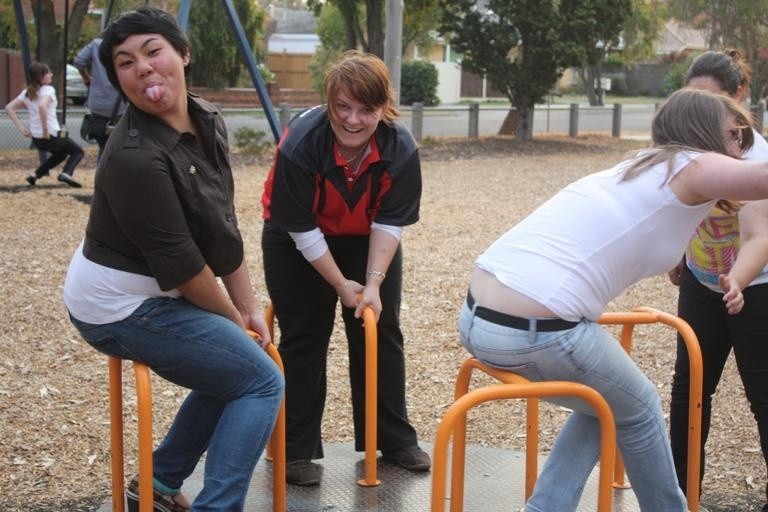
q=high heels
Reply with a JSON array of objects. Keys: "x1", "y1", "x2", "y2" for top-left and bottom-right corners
[{"x1": 127, "y1": 473, "x2": 190, "y2": 512}]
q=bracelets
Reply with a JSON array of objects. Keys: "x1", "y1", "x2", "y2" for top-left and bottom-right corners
[{"x1": 365, "y1": 269, "x2": 386, "y2": 282}]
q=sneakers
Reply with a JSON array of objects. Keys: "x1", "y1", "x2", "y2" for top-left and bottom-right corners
[
  {"x1": 286, "y1": 459, "x2": 320, "y2": 486},
  {"x1": 380, "y1": 446, "x2": 431, "y2": 469}
]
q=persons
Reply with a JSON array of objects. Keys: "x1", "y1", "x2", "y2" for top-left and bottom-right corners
[
  {"x1": 667, "y1": 47, "x2": 768, "y2": 512},
  {"x1": 456, "y1": 87, "x2": 768, "y2": 512},
  {"x1": 5, "y1": 61, "x2": 85, "y2": 189},
  {"x1": 61, "y1": 7, "x2": 287, "y2": 512},
  {"x1": 259, "y1": 48, "x2": 432, "y2": 484},
  {"x1": 73, "y1": 37, "x2": 129, "y2": 161}
]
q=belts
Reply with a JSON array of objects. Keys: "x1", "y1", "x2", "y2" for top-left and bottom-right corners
[{"x1": 466, "y1": 289, "x2": 581, "y2": 332}]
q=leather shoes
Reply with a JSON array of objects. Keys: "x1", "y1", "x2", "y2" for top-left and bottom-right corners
[
  {"x1": 26, "y1": 175, "x2": 36, "y2": 185},
  {"x1": 58, "y1": 174, "x2": 82, "y2": 187}
]
